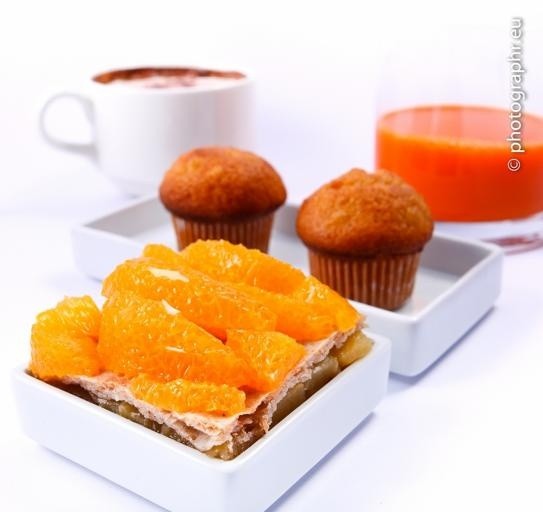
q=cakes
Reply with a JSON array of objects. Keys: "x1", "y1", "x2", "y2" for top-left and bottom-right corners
[
  {"x1": 158, "y1": 146, "x2": 287, "y2": 255},
  {"x1": 30, "y1": 239, "x2": 372, "y2": 460},
  {"x1": 295, "y1": 168, "x2": 434, "y2": 311}
]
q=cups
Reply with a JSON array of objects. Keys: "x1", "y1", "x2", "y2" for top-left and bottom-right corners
[{"x1": 38, "y1": 63, "x2": 256, "y2": 197}]
